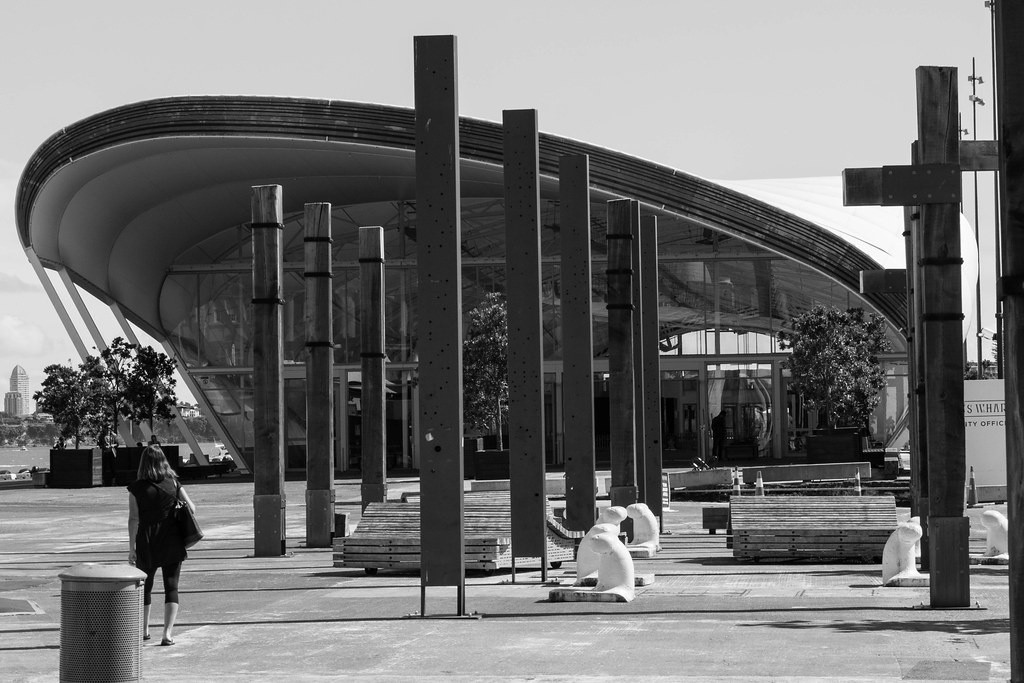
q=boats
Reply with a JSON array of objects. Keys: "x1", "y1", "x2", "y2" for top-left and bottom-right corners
[
  {"x1": 215, "y1": 443, "x2": 230, "y2": 455},
  {"x1": 20, "y1": 446, "x2": 27, "y2": 451}
]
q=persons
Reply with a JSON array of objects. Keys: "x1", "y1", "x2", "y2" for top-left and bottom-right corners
[
  {"x1": 64, "y1": 439, "x2": 67, "y2": 448},
  {"x1": 886, "y1": 415, "x2": 895, "y2": 433},
  {"x1": 53, "y1": 437, "x2": 64, "y2": 450},
  {"x1": 136, "y1": 442, "x2": 142, "y2": 447},
  {"x1": 148, "y1": 435, "x2": 160, "y2": 446},
  {"x1": 712, "y1": 410, "x2": 727, "y2": 460},
  {"x1": 104, "y1": 436, "x2": 118, "y2": 448},
  {"x1": 127, "y1": 444, "x2": 195, "y2": 646}
]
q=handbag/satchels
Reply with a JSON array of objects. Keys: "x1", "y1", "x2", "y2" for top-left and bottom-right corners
[{"x1": 175, "y1": 499, "x2": 204, "y2": 549}]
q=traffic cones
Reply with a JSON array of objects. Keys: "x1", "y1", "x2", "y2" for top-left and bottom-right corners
[
  {"x1": 731, "y1": 466, "x2": 742, "y2": 497},
  {"x1": 967, "y1": 465, "x2": 983, "y2": 509},
  {"x1": 852, "y1": 466, "x2": 863, "y2": 497},
  {"x1": 755, "y1": 470, "x2": 764, "y2": 496}
]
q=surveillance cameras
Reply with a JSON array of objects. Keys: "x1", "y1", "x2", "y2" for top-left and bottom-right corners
[{"x1": 980, "y1": 328, "x2": 996, "y2": 340}]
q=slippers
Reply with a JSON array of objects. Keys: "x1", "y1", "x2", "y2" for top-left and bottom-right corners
[
  {"x1": 161, "y1": 640, "x2": 175, "y2": 645},
  {"x1": 143, "y1": 634, "x2": 151, "y2": 639}
]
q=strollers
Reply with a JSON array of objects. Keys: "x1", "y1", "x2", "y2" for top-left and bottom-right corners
[{"x1": 690, "y1": 456, "x2": 717, "y2": 471}]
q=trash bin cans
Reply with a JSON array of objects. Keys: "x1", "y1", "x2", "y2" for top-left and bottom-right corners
[{"x1": 57, "y1": 565, "x2": 147, "y2": 683}]
